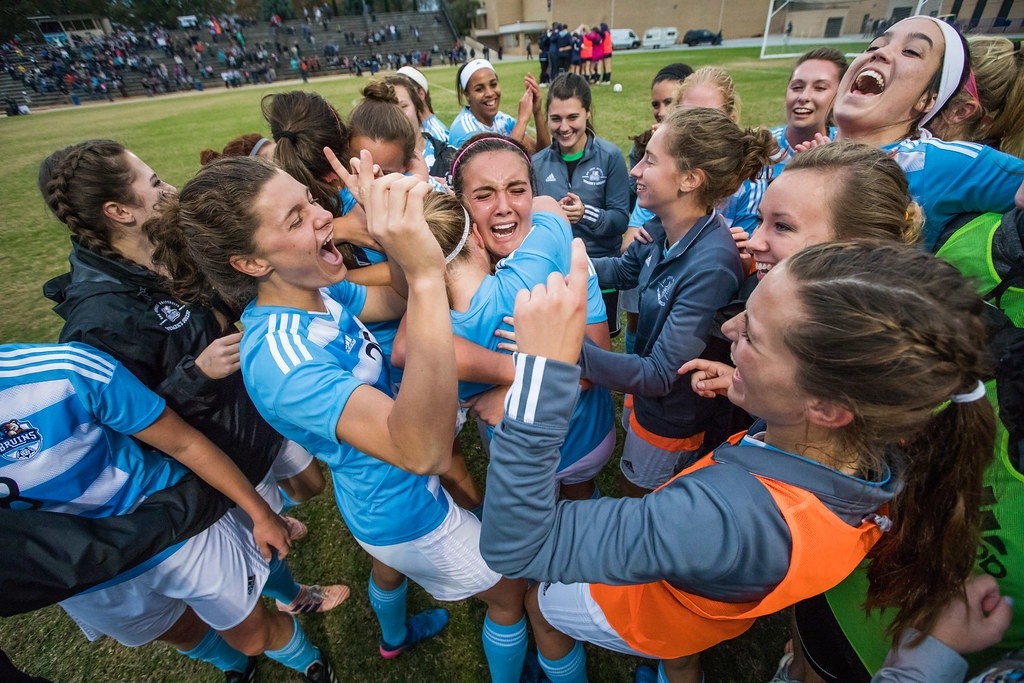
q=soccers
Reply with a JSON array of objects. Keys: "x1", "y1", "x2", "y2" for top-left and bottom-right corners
[{"x1": 613, "y1": 84, "x2": 622, "y2": 92}]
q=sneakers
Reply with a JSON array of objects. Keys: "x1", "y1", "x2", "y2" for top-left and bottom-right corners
[
  {"x1": 275, "y1": 584, "x2": 350, "y2": 615},
  {"x1": 224, "y1": 655, "x2": 257, "y2": 683},
  {"x1": 302, "y1": 647, "x2": 339, "y2": 683},
  {"x1": 279, "y1": 515, "x2": 307, "y2": 539},
  {"x1": 379, "y1": 608, "x2": 448, "y2": 660}
]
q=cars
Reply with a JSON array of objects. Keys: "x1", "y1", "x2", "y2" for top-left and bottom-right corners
[{"x1": 682, "y1": 28, "x2": 718, "y2": 47}]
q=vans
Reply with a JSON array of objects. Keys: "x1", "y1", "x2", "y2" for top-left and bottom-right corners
[
  {"x1": 609, "y1": 28, "x2": 641, "y2": 50},
  {"x1": 643, "y1": 26, "x2": 677, "y2": 48}
]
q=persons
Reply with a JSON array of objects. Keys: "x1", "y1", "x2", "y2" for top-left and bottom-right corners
[
  {"x1": 0, "y1": 0, "x2": 475, "y2": 117},
  {"x1": 478, "y1": 14, "x2": 1024, "y2": 683},
  {"x1": 0, "y1": 140, "x2": 350, "y2": 682},
  {"x1": 141, "y1": 59, "x2": 629, "y2": 683}
]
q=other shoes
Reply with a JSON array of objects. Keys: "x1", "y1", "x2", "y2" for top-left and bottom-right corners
[
  {"x1": 635, "y1": 665, "x2": 658, "y2": 683},
  {"x1": 519, "y1": 652, "x2": 540, "y2": 683}
]
